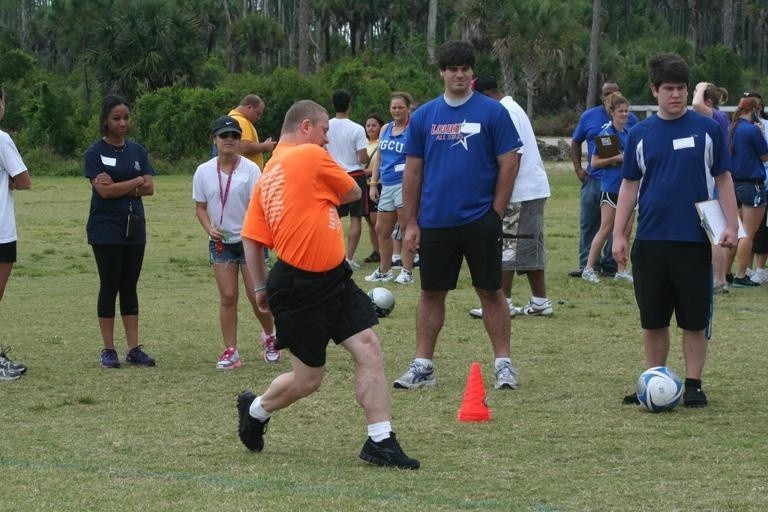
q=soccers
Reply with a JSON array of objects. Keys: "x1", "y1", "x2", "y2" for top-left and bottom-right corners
[
  {"x1": 367, "y1": 287, "x2": 395, "y2": 318},
  {"x1": 637, "y1": 365, "x2": 683, "y2": 413}
]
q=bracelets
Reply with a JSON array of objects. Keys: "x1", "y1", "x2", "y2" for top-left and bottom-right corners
[{"x1": 135, "y1": 187, "x2": 139, "y2": 197}]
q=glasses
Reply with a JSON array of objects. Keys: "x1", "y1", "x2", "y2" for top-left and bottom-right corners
[{"x1": 215, "y1": 133, "x2": 240, "y2": 140}]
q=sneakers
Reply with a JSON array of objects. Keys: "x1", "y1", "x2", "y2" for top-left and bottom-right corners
[
  {"x1": 393, "y1": 360, "x2": 436, "y2": 389},
  {"x1": 0, "y1": 345, "x2": 27, "y2": 374},
  {"x1": 215, "y1": 347, "x2": 241, "y2": 369},
  {"x1": 358, "y1": 431, "x2": 420, "y2": 471},
  {"x1": 99, "y1": 348, "x2": 120, "y2": 368},
  {"x1": 623, "y1": 392, "x2": 640, "y2": 406},
  {"x1": 712, "y1": 267, "x2": 768, "y2": 294},
  {"x1": 515, "y1": 299, "x2": 554, "y2": 317},
  {"x1": 236, "y1": 390, "x2": 271, "y2": 453},
  {"x1": 262, "y1": 330, "x2": 281, "y2": 364},
  {"x1": 349, "y1": 250, "x2": 420, "y2": 286},
  {"x1": 568, "y1": 269, "x2": 633, "y2": 286},
  {"x1": 470, "y1": 298, "x2": 516, "y2": 319},
  {"x1": 124, "y1": 344, "x2": 156, "y2": 367},
  {"x1": 682, "y1": 383, "x2": 708, "y2": 408},
  {"x1": 0, "y1": 364, "x2": 20, "y2": 380}
]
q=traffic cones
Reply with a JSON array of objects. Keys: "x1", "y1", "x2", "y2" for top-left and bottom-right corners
[{"x1": 457, "y1": 361, "x2": 490, "y2": 422}]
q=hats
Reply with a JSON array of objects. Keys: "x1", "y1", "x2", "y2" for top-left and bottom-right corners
[
  {"x1": 470, "y1": 75, "x2": 497, "y2": 91},
  {"x1": 210, "y1": 115, "x2": 243, "y2": 137}
]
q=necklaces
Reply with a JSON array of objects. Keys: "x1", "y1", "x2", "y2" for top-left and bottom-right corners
[{"x1": 112, "y1": 144, "x2": 127, "y2": 153}]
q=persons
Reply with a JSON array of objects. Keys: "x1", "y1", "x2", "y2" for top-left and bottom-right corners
[
  {"x1": 612, "y1": 56, "x2": 738, "y2": 407},
  {"x1": 0, "y1": 88, "x2": 32, "y2": 379},
  {"x1": 325, "y1": 40, "x2": 554, "y2": 390},
  {"x1": 85, "y1": 96, "x2": 156, "y2": 369},
  {"x1": 691, "y1": 81, "x2": 767, "y2": 293},
  {"x1": 194, "y1": 96, "x2": 280, "y2": 370},
  {"x1": 237, "y1": 101, "x2": 420, "y2": 469},
  {"x1": 568, "y1": 79, "x2": 641, "y2": 283}
]
